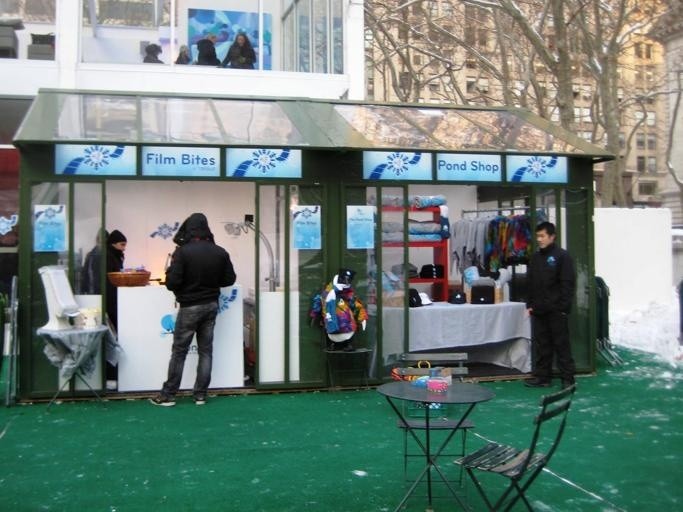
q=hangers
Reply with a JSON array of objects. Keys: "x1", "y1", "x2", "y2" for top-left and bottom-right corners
[{"x1": 460, "y1": 209, "x2": 545, "y2": 217}]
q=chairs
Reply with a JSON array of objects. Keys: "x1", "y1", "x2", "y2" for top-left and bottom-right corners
[
  {"x1": 453, "y1": 382, "x2": 577, "y2": 512},
  {"x1": 397, "y1": 352, "x2": 475, "y2": 511},
  {"x1": 322, "y1": 302, "x2": 372, "y2": 394}
]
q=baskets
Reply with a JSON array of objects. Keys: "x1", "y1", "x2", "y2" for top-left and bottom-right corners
[{"x1": 107, "y1": 270, "x2": 150, "y2": 286}]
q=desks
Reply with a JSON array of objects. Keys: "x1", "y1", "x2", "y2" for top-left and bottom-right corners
[
  {"x1": 36, "y1": 324, "x2": 111, "y2": 412},
  {"x1": 375, "y1": 378, "x2": 496, "y2": 512},
  {"x1": 365, "y1": 300, "x2": 533, "y2": 373}
]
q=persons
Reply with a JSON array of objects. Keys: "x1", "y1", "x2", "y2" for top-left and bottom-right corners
[
  {"x1": 523, "y1": 222, "x2": 575, "y2": 392},
  {"x1": 175, "y1": 45, "x2": 190, "y2": 64},
  {"x1": 82, "y1": 231, "x2": 127, "y2": 391},
  {"x1": 216, "y1": 34, "x2": 257, "y2": 68},
  {"x1": 143, "y1": 44, "x2": 165, "y2": 63},
  {"x1": 151, "y1": 212, "x2": 237, "y2": 406},
  {"x1": 197, "y1": 36, "x2": 221, "y2": 65}
]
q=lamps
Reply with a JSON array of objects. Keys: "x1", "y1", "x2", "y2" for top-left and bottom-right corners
[{"x1": 224, "y1": 221, "x2": 275, "y2": 291}]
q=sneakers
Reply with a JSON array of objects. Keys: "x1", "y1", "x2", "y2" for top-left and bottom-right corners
[
  {"x1": 192, "y1": 396, "x2": 205, "y2": 405},
  {"x1": 150, "y1": 396, "x2": 176, "y2": 406}
]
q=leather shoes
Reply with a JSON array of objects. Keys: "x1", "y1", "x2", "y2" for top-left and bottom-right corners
[
  {"x1": 561, "y1": 378, "x2": 575, "y2": 393},
  {"x1": 522, "y1": 377, "x2": 551, "y2": 387}
]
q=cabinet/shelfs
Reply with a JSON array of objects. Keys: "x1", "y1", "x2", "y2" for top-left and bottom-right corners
[
  {"x1": 376, "y1": 203, "x2": 449, "y2": 303},
  {"x1": 244, "y1": 288, "x2": 299, "y2": 383}
]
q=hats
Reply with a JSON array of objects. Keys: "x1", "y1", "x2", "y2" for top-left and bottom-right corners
[
  {"x1": 447, "y1": 292, "x2": 465, "y2": 304},
  {"x1": 339, "y1": 269, "x2": 355, "y2": 278},
  {"x1": 417, "y1": 292, "x2": 432, "y2": 305},
  {"x1": 108, "y1": 230, "x2": 125, "y2": 244}
]
